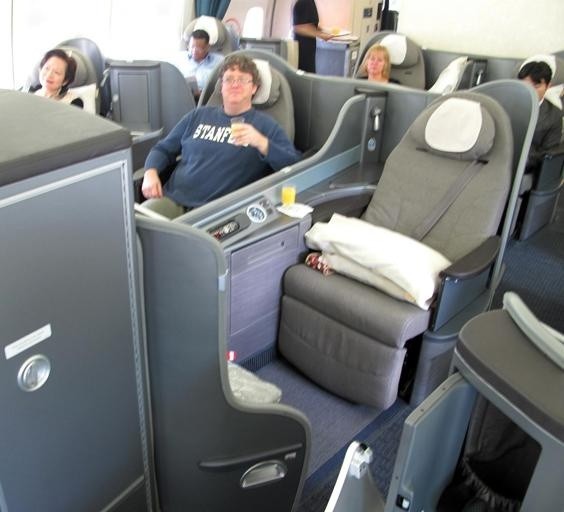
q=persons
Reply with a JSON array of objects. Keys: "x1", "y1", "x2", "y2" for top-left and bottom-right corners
[
  {"x1": 290, "y1": 0, "x2": 335, "y2": 74},
  {"x1": 356, "y1": 43, "x2": 404, "y2": 85},
  {"x1": 168, "y1": 28, "x2": 225, "y2": 106},
  {"x1": 518, "y1": 60, "x2": 564, "y2": 172},
  {"x1": 22, "y1": 47, "x2": 85, "y2": 108},
  {"x1": 141, "y1": 54, "x2": 304, "y2": 220}
]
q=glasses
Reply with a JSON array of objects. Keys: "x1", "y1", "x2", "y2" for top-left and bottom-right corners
[{"x1": 224, "y1": 77, "x2": 253, "y2": 85}]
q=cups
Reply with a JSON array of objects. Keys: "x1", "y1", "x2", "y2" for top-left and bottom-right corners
[
  {"x1": 281, "y1": 181, "x2": 296, "y2": 208},
  {"x1": 230, "y1": 117, "x2": 245, "y2": 142}
]
